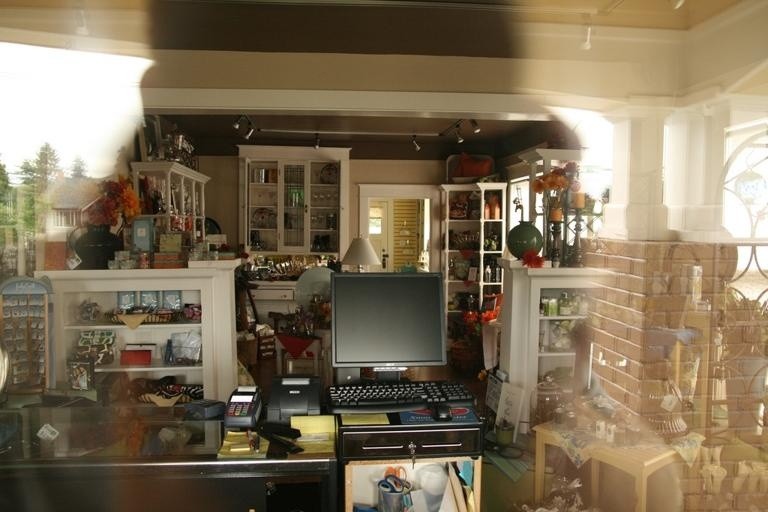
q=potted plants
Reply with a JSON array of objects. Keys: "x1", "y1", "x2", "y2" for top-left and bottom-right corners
[{"x1": 716, "y1": 287, "x2": 768, "y2": 432}]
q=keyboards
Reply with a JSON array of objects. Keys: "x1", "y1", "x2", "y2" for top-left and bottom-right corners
[{"x1": 325, "y1": 379, "x2": 477, "y2": 414}]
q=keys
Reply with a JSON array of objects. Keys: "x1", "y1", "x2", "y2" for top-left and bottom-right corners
[{"x1": 410, "y1": 441, "x2": 416, "y2": 467}]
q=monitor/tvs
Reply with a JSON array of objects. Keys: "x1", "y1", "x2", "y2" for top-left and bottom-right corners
[{"x1": 331, "y1": 272, "x2": 447, "y2": 384}]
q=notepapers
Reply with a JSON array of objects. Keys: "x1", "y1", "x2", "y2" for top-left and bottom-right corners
[{"x1": 230, "y1": 444, "x2": 251, "y2": 452}]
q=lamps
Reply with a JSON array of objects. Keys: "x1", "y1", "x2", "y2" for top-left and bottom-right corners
[
  {"x1": 441, "y1": 118, "x2": 481, "y2": 145},
  {"x1": 258, "y1": 129, "x2": 441, "y2": 152},
  {"x1": 233, "y1": 114, "x2": 256, "y2": 140},
  {"x1": 71, "y1": 8, "x2": 94, "y2": 44},
  {"x1": 579, "y1": 20, "x2": 593, "y2": 52}
]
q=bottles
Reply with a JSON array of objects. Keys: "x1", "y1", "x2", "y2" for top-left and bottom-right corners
[
  {"x1": 507, "y1": 221, "x2": 545, "y2": 259},
  {"x1": 310, "y1": 234, "x2": 337, "y2": 252},
  {"x1": 472, "y1": 256, "x2": 502, "y2": 283},
  {"x1": 484, "y1": 194, "x2": 500, "y2": 219},
  {"x1": 542, "y1": 289, "x2": 596, "y2": 316},
  {"x1": 484, "y1": 226, "x2": 502, "y2": 251},
  {"x1": 251, "y1": 231, "x2": 266, "y2": 252}
]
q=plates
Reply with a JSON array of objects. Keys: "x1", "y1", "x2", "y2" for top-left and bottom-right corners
[
  {"x1": 253, "y1": 208, "x2": 271, "y2": 228},
  {"x1": 321, "y1": 164, "x2": 336, "y2": 185}
]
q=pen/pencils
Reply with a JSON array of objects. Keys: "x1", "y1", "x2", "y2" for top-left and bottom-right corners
[
  {"x1": 379, "y1": 482, "x2": 414, "y2": 512},
  {"x1": 248, "y1": 429, "x2": 259, "y2": 453}
]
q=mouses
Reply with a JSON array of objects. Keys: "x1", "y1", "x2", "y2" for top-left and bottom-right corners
[{"x1": 433, "y1": 405, "x2": 453, "y2": 422}]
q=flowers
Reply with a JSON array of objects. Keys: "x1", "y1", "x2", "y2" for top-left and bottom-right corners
[{"x1": 514, "y1": 489, "x2": 590, "y2": 512}]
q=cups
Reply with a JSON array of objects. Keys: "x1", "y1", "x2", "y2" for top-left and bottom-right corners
[{"x1": 191, "y1": 238, "x2": 219, "y2": 260}]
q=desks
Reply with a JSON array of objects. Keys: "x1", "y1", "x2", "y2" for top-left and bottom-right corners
[
  {"x1": 0, "y1": 405, "x2": 339, "y2": 512},
  {"x1": 532, "y1": 421, "x2": 701, "y2": 512}
]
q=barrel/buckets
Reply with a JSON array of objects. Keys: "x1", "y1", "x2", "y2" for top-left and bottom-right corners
[{"x1": 378, "y1": 479, "x2": 412, "y2": 511}]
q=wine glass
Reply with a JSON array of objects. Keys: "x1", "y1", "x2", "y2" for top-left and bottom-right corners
[
  {"x1": 311, "y1": 189, "x2": 339, "y2": 206},
  {"x1": 311, "y1": 212, "x2": 326, "y2": 230}
]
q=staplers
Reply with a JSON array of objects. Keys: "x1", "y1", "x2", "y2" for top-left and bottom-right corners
[{"x1": 263, "y1": 424, "x2": 304, "y2": 455}]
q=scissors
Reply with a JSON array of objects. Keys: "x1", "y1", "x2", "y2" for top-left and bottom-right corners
[
  {"x1": 384, "y1": 467, "x2": 407, "y2": 483},
  {"x1": 378, "y1": 475, "x2": 403, "y2": 493}
]
